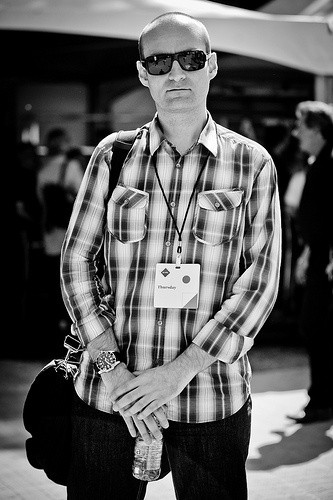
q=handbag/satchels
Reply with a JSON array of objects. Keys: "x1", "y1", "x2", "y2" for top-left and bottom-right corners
[
  {"x1": 21, "y1": 354, "x2": 84, "y2": 485},
  {"x1": 40, "y1": 180, "x2": 78, "y2": 231}
]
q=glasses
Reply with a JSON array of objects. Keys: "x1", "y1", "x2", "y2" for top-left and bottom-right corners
[{"x1": 141, "y1": 50, "x2": 212, "y2": 76}]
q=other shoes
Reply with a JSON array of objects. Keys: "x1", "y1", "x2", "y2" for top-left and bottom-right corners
[{"x1": 286, "y1": 406, "x2": 330, "y2": 425}]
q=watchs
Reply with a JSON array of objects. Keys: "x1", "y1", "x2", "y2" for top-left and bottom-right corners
[{"x1": 93, "y1": 351, "x2": 123, "y2": 374}]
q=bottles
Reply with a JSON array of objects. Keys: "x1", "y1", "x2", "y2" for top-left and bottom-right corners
[{"x1": 132, "y1": 404, "x2": 167, "y2": 481}]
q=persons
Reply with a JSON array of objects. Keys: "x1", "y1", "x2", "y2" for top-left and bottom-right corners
[
  {"x1": 280, "y1": 101, "x2": 333, "y2": 426},
  {"x1": 22, "y1": 11, "x2": 282, "y2": 500},
  {"x1": 0, "y1": 105, "x2": 307, "y2": 359}
]
